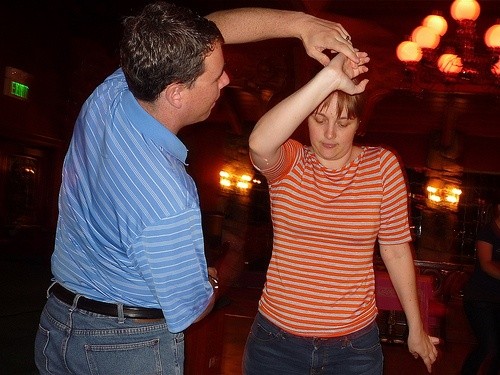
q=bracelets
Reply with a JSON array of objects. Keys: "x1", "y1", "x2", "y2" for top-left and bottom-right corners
[{"x1": 208, "y1": 274, "x2": 220, "y2": 290}]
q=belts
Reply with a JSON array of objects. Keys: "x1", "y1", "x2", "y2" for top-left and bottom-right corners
[{"x1": 50, "y1": 280, "x2": 165, "y2": 319}]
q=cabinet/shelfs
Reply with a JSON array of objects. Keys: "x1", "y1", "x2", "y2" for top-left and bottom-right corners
[{"x1": 371, "y1": 266, "x2": 443, "y2": 347}]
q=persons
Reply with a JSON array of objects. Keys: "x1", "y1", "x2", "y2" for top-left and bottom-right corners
[
  {"x1": 35, "y1": 0, "x2": 354, "y2": 375},
  {"x1": 243, "y1": 46, "x2": 440, "y2": 375},
  {"x1": 458, "y1": 202, "x2": 499, "y2": 375}
]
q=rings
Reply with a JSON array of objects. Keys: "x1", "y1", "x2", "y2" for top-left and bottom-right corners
[{"x1": 346, "y1": 36, "x2": 349, "y2": 41}]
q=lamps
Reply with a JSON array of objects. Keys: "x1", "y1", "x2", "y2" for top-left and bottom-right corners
[{"x1": 395, "y1": 0, "x2": 500, "y2": 80}]
